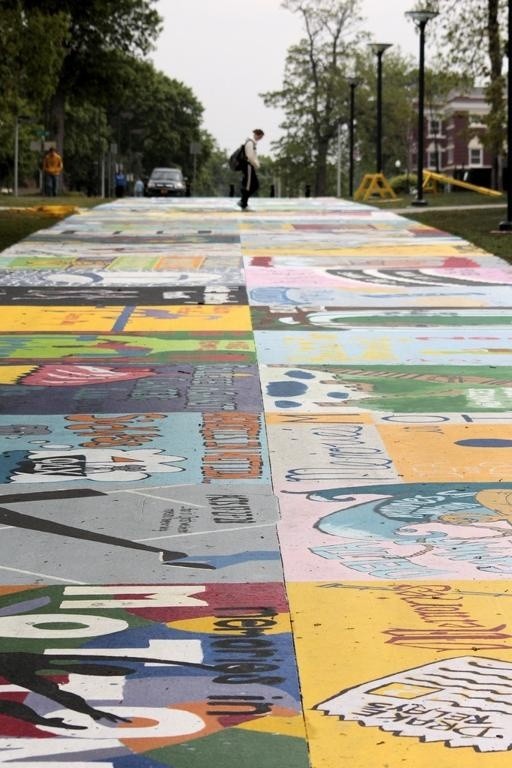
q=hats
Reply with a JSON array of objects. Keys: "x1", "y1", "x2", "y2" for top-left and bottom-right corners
[{"x1": 252, "y1": 127, "x2": 264, "y2": 135}]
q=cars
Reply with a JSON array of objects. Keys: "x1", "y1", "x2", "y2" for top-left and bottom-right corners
[{"x1": 145, "y1": 167, "x2": 189, "y2": 197}]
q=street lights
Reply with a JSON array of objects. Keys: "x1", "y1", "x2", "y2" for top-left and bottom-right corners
[
  {"x1": 368, "y1": 43, "x2": 392, "y2": 196},
  {"x1": 342, "y1": 74, "x2": 364, "y2": 196},
  {"x1": 405, "y1": 10, "x2": 437, "y2": 208}
]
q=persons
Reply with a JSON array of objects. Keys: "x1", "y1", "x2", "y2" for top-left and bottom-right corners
[
  {"x1": 114, "y1": 167, "x2": 128, "y2": 199},
  {"x1": 86, "y1": 154, "x2": 100, "y2": 198},
  {"x1": 133, "y1": 177, "x2": 145, "y2": 196},
  {"x1": 236, "y1": 129, "x2": 265, "y2": 212},
  {"x1": 42, "y1": 146, "x2": 64, "y2": 198}
]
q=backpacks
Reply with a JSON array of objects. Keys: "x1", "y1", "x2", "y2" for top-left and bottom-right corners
[{"x1": 228, "y1": 138, "x2": 255, "y2": 172}]
